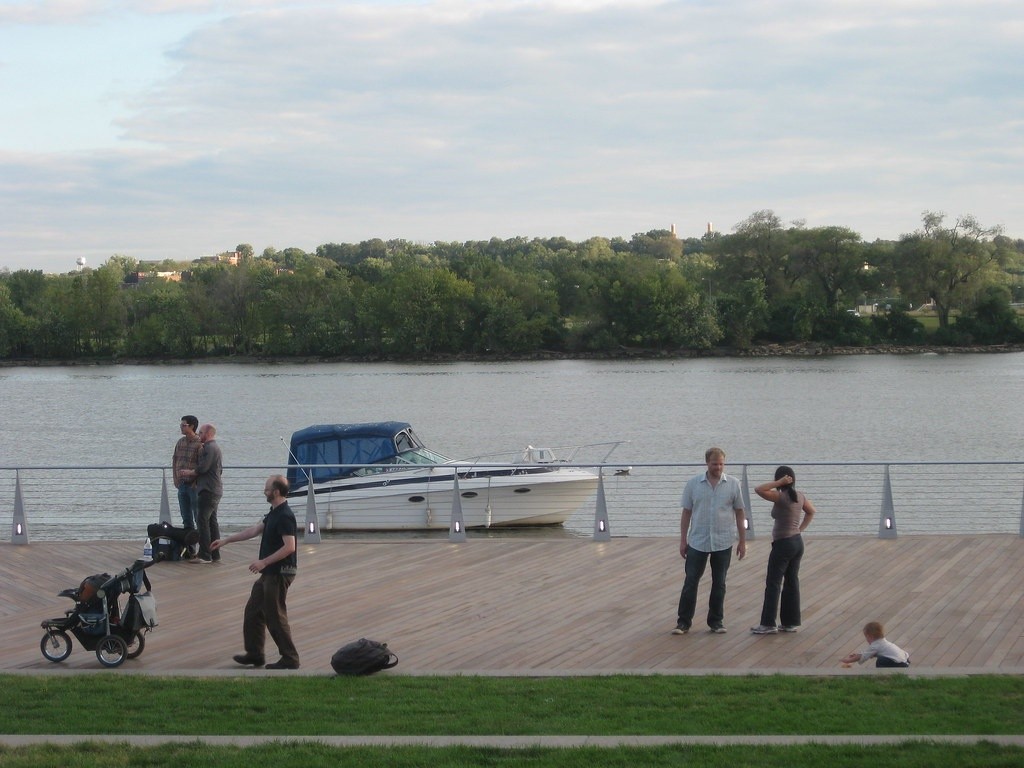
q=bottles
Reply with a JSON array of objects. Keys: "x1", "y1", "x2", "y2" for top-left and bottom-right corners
[{"x1": 144, "y1": 539, "x2": 152, "y2": 559}]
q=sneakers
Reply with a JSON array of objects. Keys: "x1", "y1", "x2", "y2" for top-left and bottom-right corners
[
  {"x1": 750, "y1": 625, "x2": 778, "y2": 633},
  {"x1": 778, "y1": 625, "x2": 797, "y2": 632},
  {"x1": 711, "y1": 626, "x2": 727, "y2": 633},
  {"x1": 671, "y1": 624, "x2": 689, "y2": 634}
]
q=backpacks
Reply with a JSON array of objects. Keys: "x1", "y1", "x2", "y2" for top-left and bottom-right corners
[{"x1": 330, "y1": 638, "x2": 399, "y2": 675}]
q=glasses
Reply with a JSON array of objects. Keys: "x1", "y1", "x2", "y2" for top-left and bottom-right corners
[{"x1": 180, "y1": 423, "x2": 191, "y2": 427}]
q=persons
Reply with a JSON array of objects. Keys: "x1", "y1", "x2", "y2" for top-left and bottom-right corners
[
  {"x1": 172, "y1": 414, "x2": 223, "y2": 565},
  {"x1": 208, "y1": 474, "x2": 301, "y2": 669},
  {"x1": 748, "y1": 465, "x2": 816, "y2": 635},
  {"x1": 672, "y1": 447, "x2": 746, "y2": 635},
  {"x1": 839, "y1": 621, "x2": 910, "y2": 668}
]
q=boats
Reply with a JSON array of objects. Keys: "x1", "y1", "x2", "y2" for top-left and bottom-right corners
[{"x1": 282, "y1": 420, "x2": 630, "y2": 532}]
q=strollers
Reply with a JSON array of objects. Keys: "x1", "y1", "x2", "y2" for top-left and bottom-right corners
[{"x1": 39, "y1": 550, "x2": 167, "y2": 668}]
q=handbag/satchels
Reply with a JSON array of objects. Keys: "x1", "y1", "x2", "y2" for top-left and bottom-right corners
[{"x1": 147, "y1": 521, "x2": 192, "y2": 562}]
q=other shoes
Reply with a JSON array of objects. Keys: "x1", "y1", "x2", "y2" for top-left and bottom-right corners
[
  {"x1": 233, "y1": 655, "x2": 265, "y2": 666},
  {"x1": 189, "y1": 554, "x2": 212, "y2": 564},
  {"x1": 212, "y1": 555, "x2": 222, "y2": 562},
  {"x1": 265, "y1": 657, "x2": 299, "y2": 670}
]
